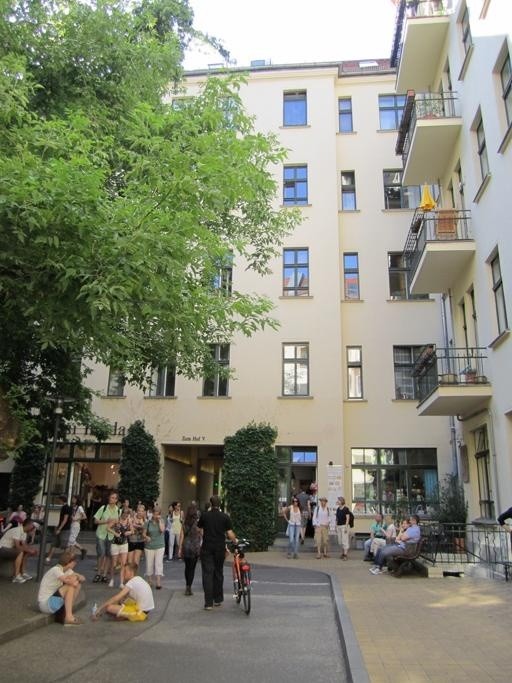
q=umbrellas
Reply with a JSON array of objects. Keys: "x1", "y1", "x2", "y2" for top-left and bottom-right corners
[{"x1": 420, "y1": 181, "x2": 437, "y2": 240}]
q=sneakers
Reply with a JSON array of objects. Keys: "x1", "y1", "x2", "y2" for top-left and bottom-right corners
[
  {"x1": 156, "y1": 585, "x2": 221, "y2": 610},
  {"x1": 81, "y1": 548, "x2": 86, "y2": 560},
  {"x1": 93, "y1": 575, "x2": 124, "y2": 589},
  {"x1": 287, "y1": 553, "x2": 348, "y2": 561},
  {"x1": 64, "y1": 616, "x2": 85, "y2": 627},
  {"x1": 22, "y1": 573, "x2": 32, "y2": 580},
  {"x1": 12, "y1": 575, "x2": 26, "y2": 584},
  {"x1": 369, "y1": 566, "x2": 389, "y2": 575}
]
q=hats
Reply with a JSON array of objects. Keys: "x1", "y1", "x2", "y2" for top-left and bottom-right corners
[
  {"x1": 338, "y1": 496, "x2": 345, "y2": 505},
  {"x1": 57, "y1": 551, "x2": 74, "y2": 566},
  {"x1": 319, "y1": 497, "x2": 327, "y2": 502}
]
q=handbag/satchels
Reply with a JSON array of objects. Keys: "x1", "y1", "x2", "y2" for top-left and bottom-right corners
[
  {"x1": 349, "y1": 512, "x2": 354, "y2": 528},
  {"x1": 115, "y1": 535, "x2": 123, "y2": 545}
]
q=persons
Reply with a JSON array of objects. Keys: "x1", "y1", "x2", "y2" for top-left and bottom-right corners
[
  {"x1": 335, "y1": 496, "x2": 350, "y2": 561},
  {"x1": 0, "y1": 484, "x2": 239, "y2": 628},
  {"x1": 362, "y1": 512, "x2": 421, "y2": 576},
  {"x1": 497, "y1": 506, "x2": 512, "y2": 549},
  {"x1": 295, "y1": 485, "x2": 312, "y2": 544},
  {"x1": 312, "y1": 497, "x2": 332, "y2": 559},
  {"x1": 283, "y1": 497, "x2": 303, "y2": 560}
]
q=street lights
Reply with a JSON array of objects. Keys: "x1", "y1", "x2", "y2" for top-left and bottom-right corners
[{"x1": 30, "y1": 392, "x2": 91, "y2": 583}]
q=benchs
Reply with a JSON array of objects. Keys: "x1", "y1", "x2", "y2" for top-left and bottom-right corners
[{"x1": 390, "y1": 537, "x2": 430, "y2": 578}]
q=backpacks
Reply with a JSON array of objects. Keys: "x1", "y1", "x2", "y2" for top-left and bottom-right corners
[{"x1": 181, "y1": 521, "x2": 201, "y2": 557}]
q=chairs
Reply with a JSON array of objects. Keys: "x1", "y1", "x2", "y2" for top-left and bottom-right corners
[{"x1": 433, "y1": 207, "x2": 458, "y2": 241}]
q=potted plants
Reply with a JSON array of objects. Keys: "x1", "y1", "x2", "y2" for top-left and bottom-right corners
[
  {"x1": 460, "y1": 365, "x2": 477, "y2": 384},
  {"x1": 428, "y1": 471, "x2": 468, "y2": 554}
]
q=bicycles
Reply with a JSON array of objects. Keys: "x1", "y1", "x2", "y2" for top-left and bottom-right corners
[{"x1": 224, "y1": 538, "x2": 257, "y2": 615}]
